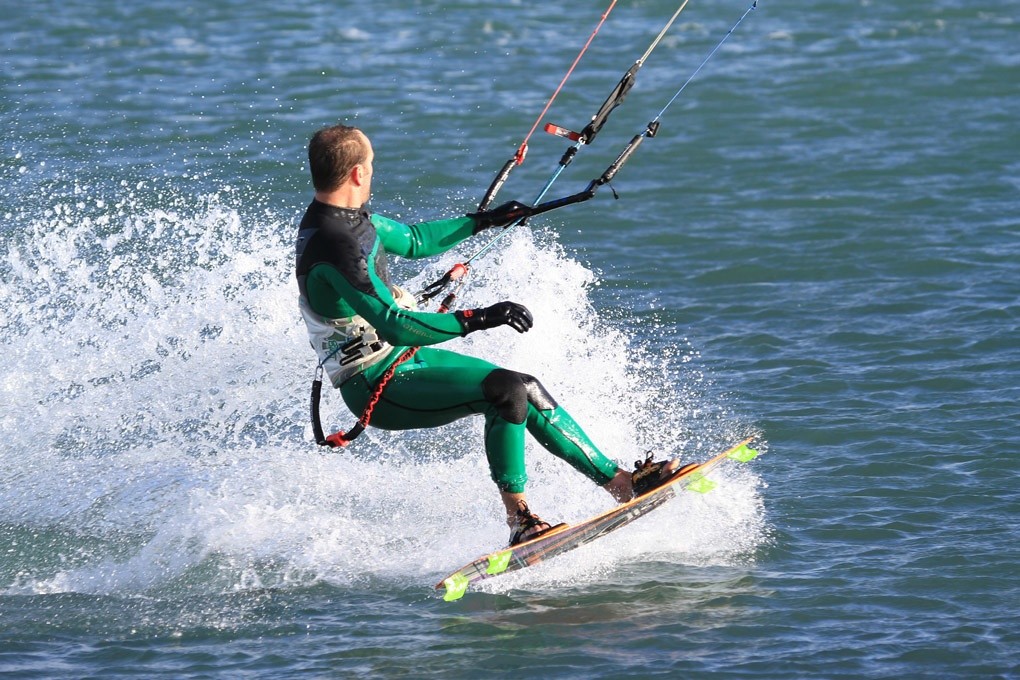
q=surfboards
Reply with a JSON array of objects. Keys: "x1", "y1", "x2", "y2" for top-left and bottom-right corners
[{"x1": 433, "y1": 439, "x2": 760, "y2": 602}]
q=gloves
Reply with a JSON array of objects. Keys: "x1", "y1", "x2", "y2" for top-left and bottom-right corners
[
  {"x1": 452, "y1": 300, "x2": 534, "y2": 339},
  {"x1": 466, "y1": 198, "x2": 535, "y2": 240}
]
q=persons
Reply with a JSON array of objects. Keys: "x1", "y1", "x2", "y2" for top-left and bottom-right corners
[{"x1": 296, "y1": 124, "x2": 681, "y2": 546}]
